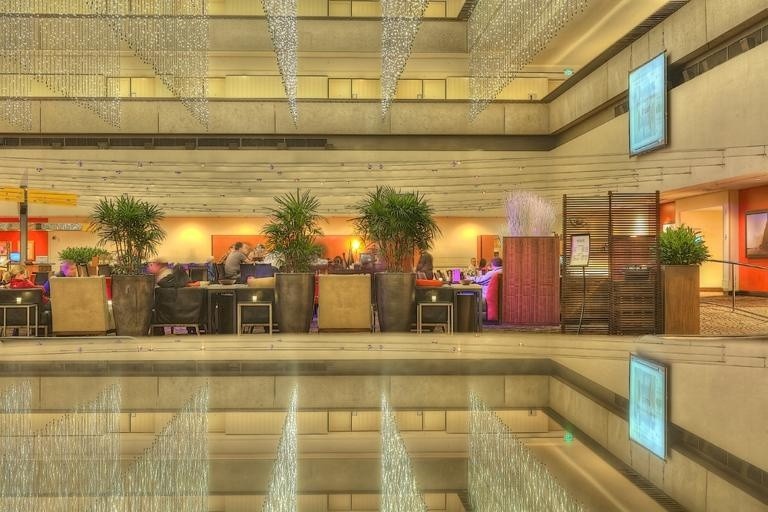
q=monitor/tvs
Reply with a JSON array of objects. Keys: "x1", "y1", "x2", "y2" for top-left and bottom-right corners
[{"x1": 9, "y1": 252, "x2": 20, "y2": 262}]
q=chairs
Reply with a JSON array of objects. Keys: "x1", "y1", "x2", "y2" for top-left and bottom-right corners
[
  {"x1": 152, "y1": 287, "x2": 207, "y2": 335},
  {"x1": 46, "y1": 274, "x2": 119, "y2": 336},
  {"x1": 315, "y1": 273, "x2": 373, "y2": 333},
  {"x1": 0, "y1": 252, "x2": 488, "y2": 300}
]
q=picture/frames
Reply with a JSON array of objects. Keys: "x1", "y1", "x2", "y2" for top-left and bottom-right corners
[{"x1": 744, "y1": 208, "x2": 768, "y2": 260}]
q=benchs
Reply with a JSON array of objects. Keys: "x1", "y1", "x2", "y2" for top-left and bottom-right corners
[{"x1": 1, "y1": 291, "x2": 50, "y2": 336}]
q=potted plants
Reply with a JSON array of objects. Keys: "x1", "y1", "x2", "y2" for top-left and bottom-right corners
[
  {"x1": 84, "y1": 190, "x2": 167, "y2": 337},
  {"x1": 345, "y1": 183, "x2": 442, "y2": 332},
  {"x1": 649, "y1": 224, "x2": 710, "y2": 336},
  {"x1": 256, "y1": 185, "x2": 332, "y2": 333}
]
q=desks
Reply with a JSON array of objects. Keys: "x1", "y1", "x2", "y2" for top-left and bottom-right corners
[{"x1": 447, "y1": 283, "x2": 482, "y2": 334}]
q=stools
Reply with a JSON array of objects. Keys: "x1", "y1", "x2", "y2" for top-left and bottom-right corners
[
  {"x1": 416, "y1": 299, "x2": 453, "y2": 337},
  {"x1": 235, "y1": 302, "x2": 273, "y2": 334},
  {"x1": 1, "y1": 302, "x2": 37, "y2": 337}
]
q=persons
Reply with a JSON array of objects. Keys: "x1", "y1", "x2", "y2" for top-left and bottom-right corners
[
  {"x1": 2, "y1": 264, "x2": 52, "y2": 311},
  {"x1": 156, "y1": 262, "x2": 191, "y2": 288},
  {"x1": 153, "y1": 261, "x2": 169, "y2": 286},
  {"x1": 222, "y1": 242, "x2": 265, "y2": 279},
  {"x1": 43, "y1": 260, "x2": 78, "y2": 297},
  {"x1": 466, "y1": 250, "x2": 502, "y2": 302},
  {"x1": 417, "y1": 247, "x2": 434, "y2": 281}
]
